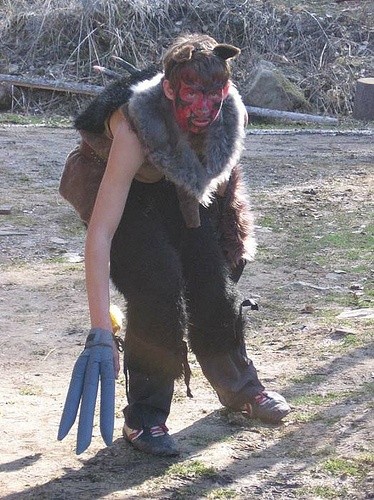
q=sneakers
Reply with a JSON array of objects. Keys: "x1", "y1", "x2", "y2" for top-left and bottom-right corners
[
  {"x1": 122, "y1": 420, "x2": 177, "y2": 455},
  {"x1": 240, "y1": 388, "x2": 290, "y2": 420}
]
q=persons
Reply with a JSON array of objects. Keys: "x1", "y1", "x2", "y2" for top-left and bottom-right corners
[{"x1": 58, "y1": 34, "x2": 290, "y2": 457}]
